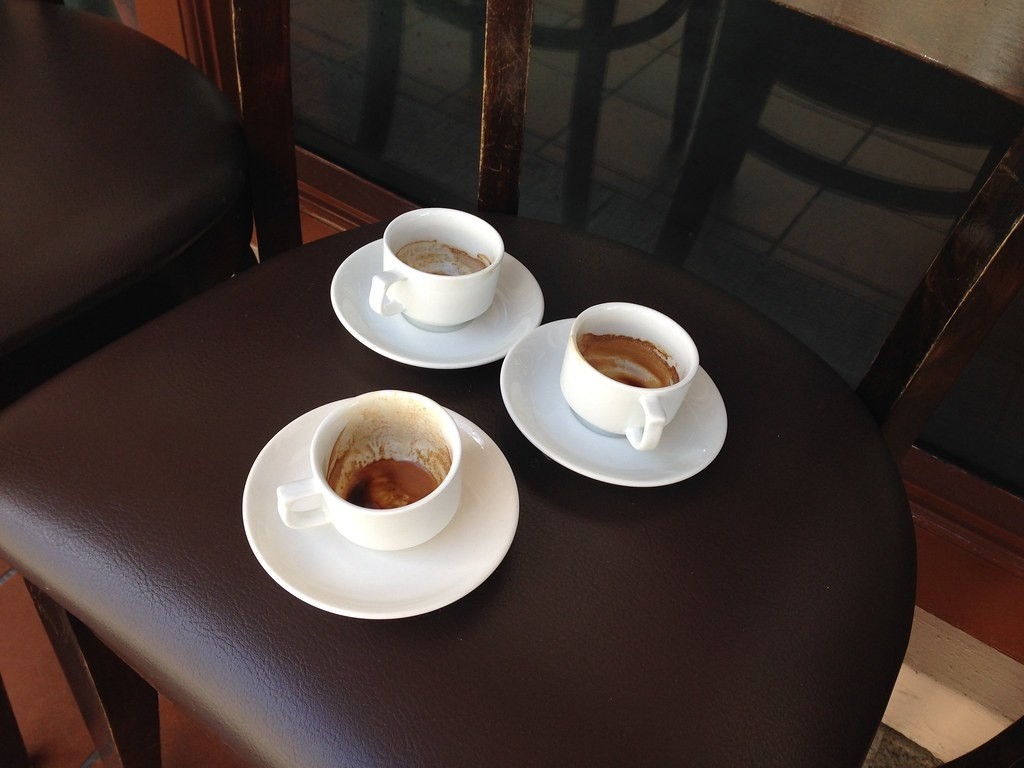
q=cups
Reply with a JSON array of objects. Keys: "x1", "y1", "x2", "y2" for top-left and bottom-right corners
[
  {"x1": 559, "y1": 301, "x2": 699, "y2": 453},
  {"x1": 276, "y1": 389, "x2": 462, "y2": 551},
  {"x1": 368, "y1": 207, "x2": 505, "y2": 327}
]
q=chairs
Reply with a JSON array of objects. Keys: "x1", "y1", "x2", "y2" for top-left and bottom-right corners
[
  {"x1": 0, "y1": 0, "x2": 294, "y2": 768},
  {"x1": 0, "y1": 2, "x2": 1022, "y2": 768},
  {"x1": 359, "y1": 0, "x2": 1024, "y2": 270}
]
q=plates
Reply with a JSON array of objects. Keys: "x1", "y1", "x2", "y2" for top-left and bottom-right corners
[
  {"x1": 501, "y1": 319, "x2": 728, "y2": 487},
  {"x1": 242, "y1": 396, "x2": 519, "y2": 619},
  {"x1": 330, "y1": 237, "x2": 544, "y2": 369}
]
